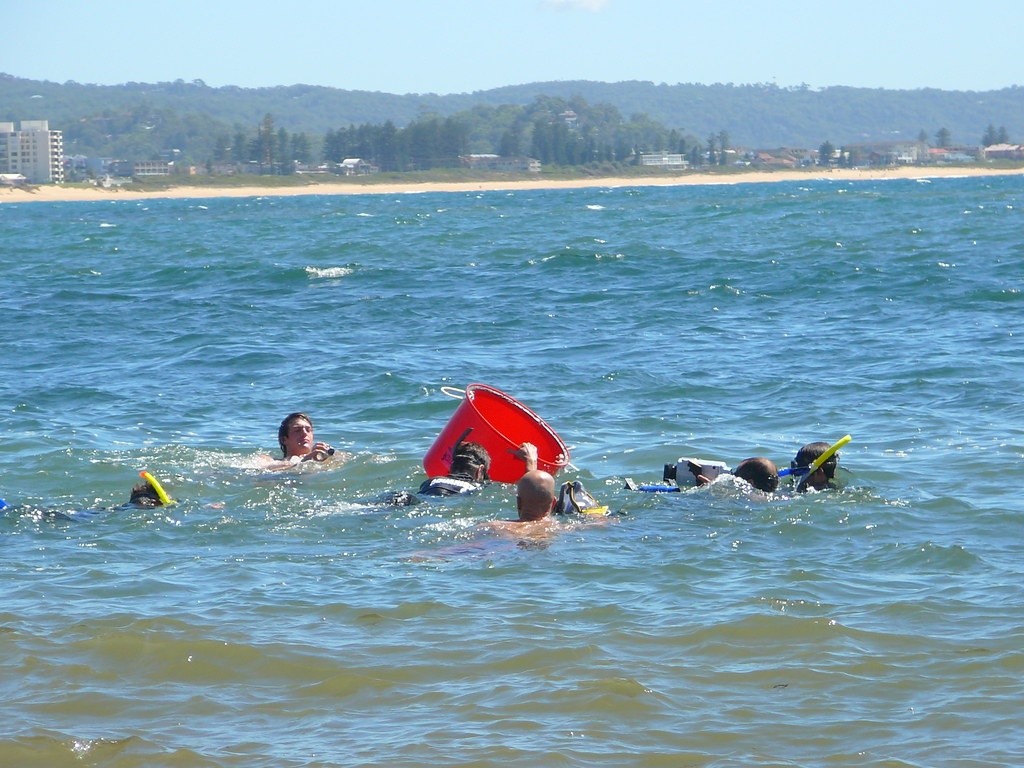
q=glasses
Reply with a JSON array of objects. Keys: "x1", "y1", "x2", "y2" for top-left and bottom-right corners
[
  {"x1": 791, "y1": 459, "x2": 837, "y2": 477},
  {"x1": 451, "y1": 451, "x2": 489, "y2": 480}
]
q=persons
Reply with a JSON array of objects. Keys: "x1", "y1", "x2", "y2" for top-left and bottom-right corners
[
  {"x1": 260, "y1": 413, "x2": 329, "y2": 462},
  {"x1": 519, "y1": 441, "x2": 609, "y2": 515},
  {"x1": 378, "y1": 441, "x2": 490, "y2": 506},
  {"x1": 733, "y1": 457, "x2": 778, "y2": 492},
  {"x1": 790, "y1": 442, "x2": 836, "y2": 492},
  {"x1": 475, "y1": 470, "x2": 558, "y2": 525},
  {"x1": 130, "y1": 481, "x2": 162, "y2": 506}
]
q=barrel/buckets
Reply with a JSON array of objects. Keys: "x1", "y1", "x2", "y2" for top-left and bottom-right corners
[{"x1": 423, "y1": 384, "x2": 569, "y2": 484}]
